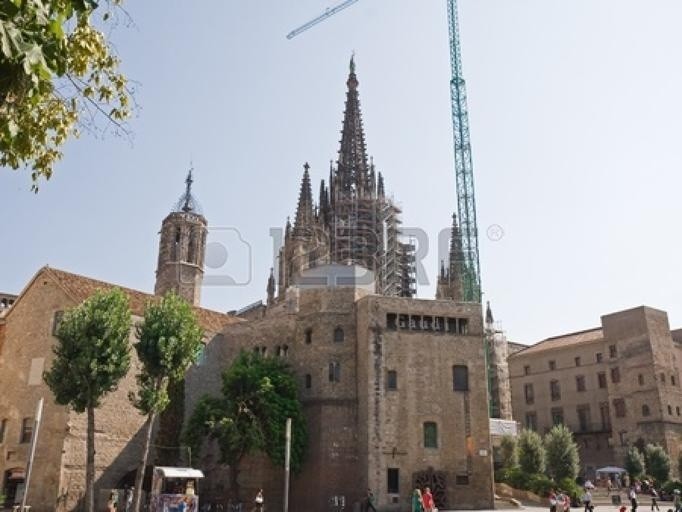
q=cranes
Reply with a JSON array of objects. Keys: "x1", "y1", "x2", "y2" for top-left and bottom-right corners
[{"x1": 284, "y1": 0, "x2": 483, "y2": 302}]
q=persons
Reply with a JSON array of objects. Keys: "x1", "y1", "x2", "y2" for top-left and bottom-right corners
[
  {"x1": 553, "y1": 487, "x2": 564, "y2": 512},
  {"x1": 604, "y1": 470, "x2": 682, "y2": 512},
  {"x1": 120, "y1": 480, "x2": 135, "y2": 510},
  {"x1": 411, "y1": 487, "x2": 424, "y2": 511},
  {"x1": 255, "y1": 487, "x2": 265, "y2": 511},
  {"x1": 547, "y1": 487, "x2": 557, "y2": 511},
  {"x1": 561, "y1": 490, "x2": 571, "y2": 512},
  {"x1": 177, "y1": 496, "x2": 192, "y2": 511},
  {"x1": 580, "y1": 486, "x2": 593, "y2": 511},
  {"x1": 363, "y1": 488, "x2": 377, "y2": 511},
  {"x1": 107, "y1": 491, "x2": 119, "y2": 512},
  {"x1": 420, "y1": 486, "x2": 436, "y2": 512}
]
